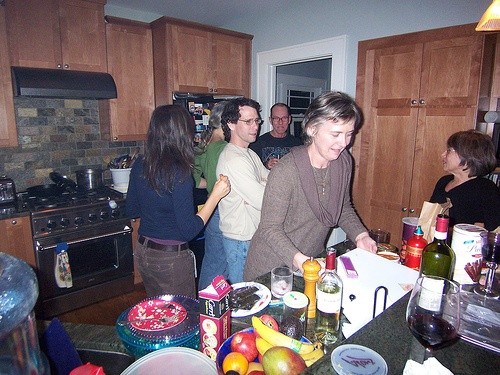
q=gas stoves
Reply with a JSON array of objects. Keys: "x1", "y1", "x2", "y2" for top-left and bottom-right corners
[{"x1": 16, "y1": 184, "x2": 123, "y2": 217}]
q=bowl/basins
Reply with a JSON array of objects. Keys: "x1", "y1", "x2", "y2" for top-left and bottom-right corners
[
  {"x1": 216, "y1": 326, "x2": 317, "y2": 375},
  {"x1": 375, "y1": 243, "x2": 397, "y2": 253},
  {"x1": 376, "y1": 251, "x2": 401, "y2": 264}
]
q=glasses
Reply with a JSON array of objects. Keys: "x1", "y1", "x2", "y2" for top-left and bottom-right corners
[
  {"x1": 271, "y1": 117, "x2": 289, "y2": 122},
  {"x1": 446, "y1": 147, "x2": 455, "y2": 154},
  {"x1": 237, "y1": 117, "x2": 262, "y2": 125}
]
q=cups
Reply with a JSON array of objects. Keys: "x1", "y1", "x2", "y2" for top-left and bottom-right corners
[
  {"x1": 271, "y1": 267, "x2": 294, "y2": 299},
  {"x1": 370, "y1": 228, "x2": 390, "y2": 244},
  {"x1": 400, "y1": 216, "x2": 420, "y2": 265},
  {"x1": 282, "y1": 291, "x2": 309, "y2": 337}
]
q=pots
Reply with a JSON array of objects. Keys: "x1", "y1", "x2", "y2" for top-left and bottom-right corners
[
  {"x1": 26, "y1": 182, "x2": 65, "y2": 200},
  {"x1": 75, "y1": 169, "x2": 103, "y2": 192}
]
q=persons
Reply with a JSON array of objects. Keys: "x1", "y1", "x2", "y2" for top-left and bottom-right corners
[
  {"x1": 243, "y1": 91, "x2": 377, "y2": 282},
  {"x1": 216, "y1": 97, "x2": 271, "y2": 284},
  {"x1": 428, "y1": 129, "x2": 500, "y2": 248},
  {"x1": 126, "y1": 105, "x2": 231, "y2": 299},
  {"x1": 248, "y1": 102, "x2": 304, "y2": 171},
  {"x1": 192, "y1": 100, "x2": 230, "y2": 292}
]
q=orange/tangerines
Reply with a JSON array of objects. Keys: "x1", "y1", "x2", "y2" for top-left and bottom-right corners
[
  {"x1": 223, "y1": 352, "x2": 249, "y2": 375},
  {"x1": 244, "y1": 362, "x2": 264, "y2": 375}
]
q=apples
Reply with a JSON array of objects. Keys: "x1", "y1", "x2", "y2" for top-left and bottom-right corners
[
  {"x1": 260, "y1": 314, "x2": 278, "y2": 330},
  {"x1": 247, "y1": 370, "x2": 264, "y2": 375},
  {"x1": 262, "y1": 346, "x2": 307, "y2": 375},
  {"x1": 231, "y1": 333, "x2": 258, "y2": 362}
]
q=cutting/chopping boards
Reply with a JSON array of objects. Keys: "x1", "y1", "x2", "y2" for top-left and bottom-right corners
[{"x1": 315, "y1": 247, "x2": 425, "y2": 342}]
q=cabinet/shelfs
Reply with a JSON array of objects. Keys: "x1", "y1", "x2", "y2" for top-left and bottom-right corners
[
  {"x1": 153, "y1": 16, "x2": 254, "y2": 108},
  {"x1": 0, "y1": 0, "x2": 107, "y2": 148},
  {"x1": 352, "y1": 21, "x2": 498, "y2": 250},
  {"x1": 0, "y1": 216, "x2": 36, "y2": 269},
  {"x1": 98, "y1": 15, "x2": 156, "y2": 144}
]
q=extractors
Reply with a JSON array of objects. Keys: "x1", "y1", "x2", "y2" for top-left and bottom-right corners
[{"x1": 11, "y1": 66, "x2": 118, "y2": 100}]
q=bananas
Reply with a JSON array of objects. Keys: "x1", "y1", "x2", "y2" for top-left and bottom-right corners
[{"x1": 252, "y1": 315, "x2": 322, "y2": 356}]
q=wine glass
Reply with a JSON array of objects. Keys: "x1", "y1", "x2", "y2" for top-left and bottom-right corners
[
  {"x1": 405, "y1": 275, "x2": 461, "y2": 365},
  {"x1": 474, "y1": 230, "x2": 500, "y2": 299}
]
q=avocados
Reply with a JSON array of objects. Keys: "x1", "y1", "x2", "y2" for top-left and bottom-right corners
[{"x1": 279, "y1": 315, "x2": 303, "y2": 341}]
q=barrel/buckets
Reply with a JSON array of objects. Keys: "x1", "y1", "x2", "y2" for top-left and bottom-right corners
[
  {"x1": 109, "y1": 167, "x2": 132, "y2": 188},
  {"x1": 450, "y1": 223, "x2": 487, "y2": 285}
]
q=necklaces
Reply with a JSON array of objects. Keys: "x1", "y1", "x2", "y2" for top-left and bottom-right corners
[{"x1": 313, "y1": 161, "x2": 329, "y2": 195}]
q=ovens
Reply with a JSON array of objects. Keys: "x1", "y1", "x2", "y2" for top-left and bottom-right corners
[{"x1": 31, "y1": 199, "x2": 135, "y2": 320}]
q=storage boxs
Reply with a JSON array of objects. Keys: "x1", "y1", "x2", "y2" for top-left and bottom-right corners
[{"x1": 198, "y1": 276, "x2": 232, "y2": 361}]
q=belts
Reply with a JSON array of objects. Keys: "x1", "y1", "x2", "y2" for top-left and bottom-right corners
[{"x1": 138, "y1": 235, "x2": 189, "y2": 252}]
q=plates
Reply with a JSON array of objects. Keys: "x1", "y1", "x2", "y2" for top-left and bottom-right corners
[
  {"x1": 230, "y1": 281, "x2": 272, "y2": 318},
  {"x1": 119, "y1": 346, "x2": 219, "y2": 375},
  {"x1": 114, "y1": 293, "x2": 202, "y2": 359}
]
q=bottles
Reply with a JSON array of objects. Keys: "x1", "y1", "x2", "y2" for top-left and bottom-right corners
[{"x1": 314, "y1": 214, "x2": 493, "y2": 344}]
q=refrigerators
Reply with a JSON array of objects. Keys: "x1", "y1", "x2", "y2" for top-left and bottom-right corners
[{"x1": 172, "y1": 91, "x2": 245, "y2": 215}]
